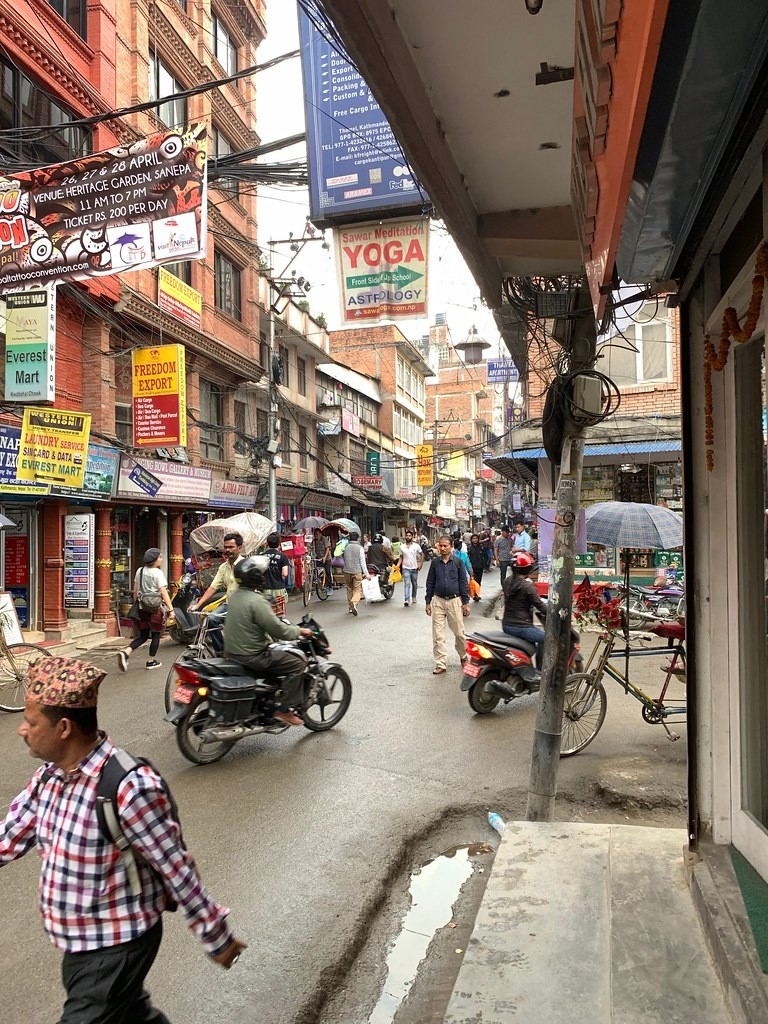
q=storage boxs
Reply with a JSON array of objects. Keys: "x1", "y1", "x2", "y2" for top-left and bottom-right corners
[{"x1": 207, "y1": 676, "x2": 256, "y2": 720}]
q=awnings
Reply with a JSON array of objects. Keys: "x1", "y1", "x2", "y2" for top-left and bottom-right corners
[{"x1": 352, "y1": 496, "x2": 423, "y2": 510}]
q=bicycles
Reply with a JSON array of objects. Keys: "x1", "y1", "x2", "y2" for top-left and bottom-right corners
[
  {"x1": 0, "y1": 601, "x2": 56, "y2": 713},
  {"x1": 163, "y1": 608, "x2": 227, "y2": 727},
  {"x1": 559, "y1": 618, "x2": 688, "y2": 757}
]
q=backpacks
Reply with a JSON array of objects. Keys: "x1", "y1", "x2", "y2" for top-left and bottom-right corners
[{"x1": 32, "y1": 749, "x2": 181, "y2": 913}]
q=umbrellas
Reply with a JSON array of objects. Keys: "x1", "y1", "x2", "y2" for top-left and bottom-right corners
[
  {"x1": 291, "y1": 516, "x2": 328, "y2": 529},
  {"x1": 582, "y1": 500, "x2": 683, "y2": 695}
]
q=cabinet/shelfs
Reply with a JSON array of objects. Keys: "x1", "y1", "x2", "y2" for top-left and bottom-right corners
[{"x1": 107, "y1": 506, "x2": 131, "y2": 603}]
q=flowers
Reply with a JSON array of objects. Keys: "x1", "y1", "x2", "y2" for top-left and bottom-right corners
[{"x1": 571, "y1": 589, "x2": 624, "y2": 643}]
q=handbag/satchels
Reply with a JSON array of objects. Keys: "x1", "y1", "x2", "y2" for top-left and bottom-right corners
[
  {"x1": 469, "y1": 580, "x2": 480, "y2": 598},
  {"x1": 362, "y1": 575, "x2": 386, "y2": 605},
  {"x1": 388, "y1": 564, "x2": 402, "y2": 583},
  {"x1": 139, "y1": 596, "x2": 162, "y2": 613}
]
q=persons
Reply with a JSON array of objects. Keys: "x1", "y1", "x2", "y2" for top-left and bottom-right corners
[
  {"x1": 396, "y1": 531, "x2": 424, "y2": 606},
  {"x1": 363, "y1": 529, "x2": 404, "y2": 592},
  {"x1": 425, "y1": 536, "x2": 471, "y2": 675},
  {"x1": 0, "y1": 657, "x2": 247, "y2": 1024},
  {"x1": 186, "y1": 532, "x2": 257, "y2": 658},
  {"x1": 419, "y1": 531, "x2": 431, "y2": 560},
  {"x1": 502, "y1": 552, "x2": 548, "y2": 671},
  {"x1": 225, "y1": 556, "x2": 315, "y2": 726},
  {"x1": 308, "y1": 528, "x2": 334, "y2": 596},
  {"x1": 342, "y1": 532, "x2": 371, "y2": 616},
  {"x1": 453, "y1": 520, "x2": 538, "y2": 616},
  {"x1": 260, "y1": 532, "x2": 289, "y2": 642},
  {"x1": 117, "y1": 548, "x2": 175, "y2": 673}
]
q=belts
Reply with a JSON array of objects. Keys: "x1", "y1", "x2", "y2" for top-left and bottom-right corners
[{"x1": 437, "y1": 593, "x2": 460, "y2": 600}]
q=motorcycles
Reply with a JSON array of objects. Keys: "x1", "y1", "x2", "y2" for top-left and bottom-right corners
[
  {"x1": 460, "y1": 609, "x2": 584, "y2": 714},
  {"x1": 366, "y1": 562, "x2": 395, "y2": 599},
  {"x1": 164, "y1": 616, "x2": 353, "y2": 765},
  {"x1": 420, "y1": 540, "x2": 434, "y2": 561}
]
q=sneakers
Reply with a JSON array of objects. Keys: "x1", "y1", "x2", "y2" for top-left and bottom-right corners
[
  {"x1": 117, "y1": 651, "x2": 129, "y2": 673},
  {"x1": 274, "y1": 710, "x2": 304, "y2": 726},
  {"x1": 146, "y1": 659, "x2": 162, "y2": 670}
]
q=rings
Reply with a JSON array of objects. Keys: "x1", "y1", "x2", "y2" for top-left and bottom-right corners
[{"x1": 230, "y1": 955, "x2": 238, "y2": 966}]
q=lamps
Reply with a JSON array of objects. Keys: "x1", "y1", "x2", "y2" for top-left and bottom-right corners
[{"x1": 664, "y1": 294, "x2": 680, "y2": 308}]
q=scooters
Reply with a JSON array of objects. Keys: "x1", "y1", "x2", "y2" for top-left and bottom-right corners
[{"x1": 163, "y1": 570, "x2": 228, "y2": 643}]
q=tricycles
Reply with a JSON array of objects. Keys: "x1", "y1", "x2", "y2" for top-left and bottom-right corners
[{"x1": 299, "y1": 518, "x2": 361, "y2": 607}]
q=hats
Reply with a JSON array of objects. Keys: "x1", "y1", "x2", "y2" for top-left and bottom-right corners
[
  {"x1": 143, "y1": 548, "x2": 160, "y2": 565},
  {"x1": 267, "y1": 532, "x2": 280, "y2": 548},
  {"x1": 24, "y1": 656, "x2": 108, "y2": 709},
  {"x1": 501, "y1": 526, "x2": 511, "y2": 533}
]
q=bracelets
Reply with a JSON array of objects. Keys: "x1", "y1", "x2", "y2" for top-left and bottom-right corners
[{"x1": 168, "y1": 610, "x2": 173, "y2": 612}]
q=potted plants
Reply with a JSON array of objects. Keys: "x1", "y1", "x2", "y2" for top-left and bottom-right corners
[{"x1": 298, "y1": 300, "x2": 310, "y2": 311}]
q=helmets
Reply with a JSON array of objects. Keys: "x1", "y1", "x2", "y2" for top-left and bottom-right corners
[
  {"x1": 372, "y1": 534, "x2": 383, "y2": 544},
  {"x1": 233, "y1": 556, "x2": 270, "y2": 588},
  {"x1": 510, "y1": 551, "x2": 535, "y2": 576}
]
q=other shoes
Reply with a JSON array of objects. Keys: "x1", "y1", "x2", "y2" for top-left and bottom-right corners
[
  {"x1": 433, "y1": 667, "x2": 445, "y2": 674},
  {"x1": 349, "y1": 602, "x2": 358, "y2": 616},
  {"x1": 473, "y1": 595, "x2": 481, "y2": 602},
  {"x1": 326, "y1": 587, "x2": 333, "y2": 596},
  {"x1": 412, "y1": 597, "x2": 417, "y2": 603},
  {"x1": 460, "y1": 656, "x2": 469, "y2": 668},
  {"x1": 403, "y1": 600, "x2": 409, "y2": 607}
]
